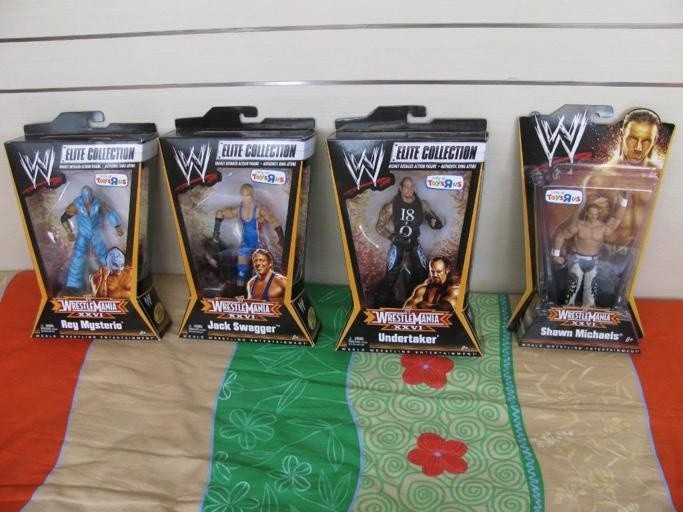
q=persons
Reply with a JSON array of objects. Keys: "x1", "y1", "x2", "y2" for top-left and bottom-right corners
[
  {"x1": 576, "y1": 108, "x2": 663, "y2": 308},
  {"x1": 370, "y1": 174, "x2": 445, "y2": 308},
  {"x1": 86, "y1": 246, "x2": 133, "y2": 301},
  {"x1": 246, "y1": 248, "x2": 288, "y2": 302},
  {"x1": 550, "y1": 190, "x2": 632, "y2": 308},
  {"x1": 401, "y1": 253, "x2": 462, "y2": 313},
  {"x1": 58, "y1": 184, "x2": 123, "y2": 298},
  {"x1": 208, "y1": 183, "x2": 286, "y2": 298}
]
